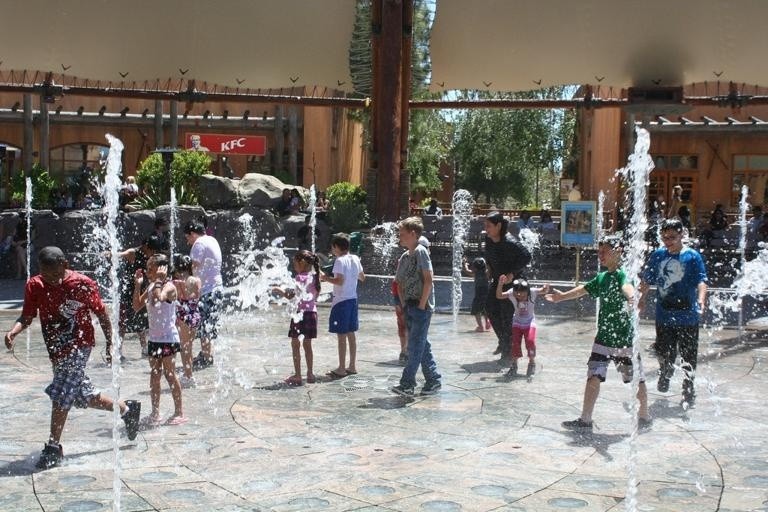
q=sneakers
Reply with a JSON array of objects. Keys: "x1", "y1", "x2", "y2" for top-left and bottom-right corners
[
  {"x1": 486, "y1": 319, "x2": 491, "y2": 329},
  {"x1": 421, "y1": 379, "x2": 442, "y2": 396},
  {"x1": 121, "y1": 398, "x2": 142, "y2": 441},
  {"x1": 476, "y1": 327, "x2": 484, "y2": 332},
  {"x1": 657, "y1": 377, "x2": 670, "y2": 392},
  {"x1": 399, "y1": 353, "x2": 408, "y2": 367},
  {"x1": 178, "y1": 377, "x2": 197, "y2": 390},
  {"x1": 35, "y1": 443, "x2": 64, "y2": 470},
  {"x1": 192, "y1": 351, "x2": 213, "y2": 372},
  {"x1": 636, "y1": 416, "x2": 654, "y2": 435},
  {"x1": 392, "y1": 384, "x2": 415, "y2": 400},
  {"x1": 493, "y1": 347, "x2": 502, "y2": 354},
  {"x1": 561, "y1": 419, "x2": 594, "y2": 435},
  {"x1": 682, "y1": 395, "x2": 697, "y2": 409}
]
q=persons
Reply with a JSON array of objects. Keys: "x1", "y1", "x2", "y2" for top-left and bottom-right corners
[
  {"x1": 139, "y1": 239, "x2": 156, "y2": 258},
  {"x1": 544, "y1": 234, "x2": 654, "y2": 433},
  {"x1": 745, "y1": 205, "x2": 763, "y2": 246},
  {"x1": 536, "y1": 209, "x2": 558, "y2": 233},
  {"x1": 89, "y1": 186, "x2": 104, "y2": 208},
  {"x1": 5, "y1": 246, "x2": 141, "y2": 471},
  {"x1": 132, "y1": 253, "x2": 187, "y2": 427},
  {"x1": 516, "y1": 210, "x2": 536, "y2": 230},
  {"x1": 57, "y1": 191, "x2": 70, "y2": 210},
  {"x1": 314, "y1": 191, "x2": 329, "y2": 209},
  {"x1": 173, "y1": 255, "x2": 202, "y2": 389},
  {"x1": 391, "y1": 234, "x2": 431, "y2": 363},
  {"x1": 666, "y1": 185, "x2": 685, "y2": 219},
  {"x1": 677, "y1": 206, "x2": 690, "y2": 227},
  {"x1": 319, "y1": 236, "x2": 365, "y2": 377},
  {"x1": 424, "y1": 199, "x2": 443, "y2": 217},
  {"x1": 388, "y1": 216, "x2": 442, "y2": 399},
  {"x1": 709, "y1": 209, "x2": 728, "y2": 248},
  {"x1": 284, "y1": 189, "x2": 304, "y2": 215},
  {"x1": 713, "y1": 205, "x2": 723, "y2": 210},
  {"x1": 568, "y1": 181, "x2": 582, "y2": 201},
  {"x1": 638, "y1": 219, "x2": 708, "y2": 409},
  {"x1": 273, "y1": 189, "x2": 291, "y2": 215},
  {"x1": 464, "y1": 257, "x2": 491, "y2": 333},
  {"x1": 11, "y1": 212, "x2": 35, "y2": 282},
  {"x1": 187, "y1": 134, "x2": 211, "y2": 153},
  {"x1": 117, "y1": 248, "x2": 150, "y2": 356},
  {"x1": 410, "y1": 198, "x2": 420, "y2": 215},
  {"x1": 496, "y1": 274, "x2": 541, "y2": 377},
  {"x1": 483, "y1": 211, "x2": 531, "y2": 366},
  {"x1": 184, "y1": 218, "x2": 223, "y2": 371},
  {"x1": 272, "y1": 250, "x2": 321, "y2": 386},
  {"x1": 124, "y1": 176, "x2": 139, "y2": 198}
]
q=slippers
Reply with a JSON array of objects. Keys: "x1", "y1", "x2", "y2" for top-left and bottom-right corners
[
  {"x1": 139, "y1": 415, "x2": 164, "y2": 426},
  {"x1": 345, "y1": 368, "x2": 357, "y2": 375},
  {"x1": 167, "y1": 415, "x2": 190, "y2": 425},
  {"x1": 285, "y1": 376, "x2": 302, "y2": 386},
  {"x1": 325, "y1": 370, "x2": 347, "y2": 378},
  {"x1": 307, "y1": 374, "x2": 316, "y2": 383}
]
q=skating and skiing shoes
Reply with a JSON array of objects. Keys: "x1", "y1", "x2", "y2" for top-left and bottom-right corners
[
  {"x1": 526, "y1": 362, "x2": 535, "y2": 383},
  {"x1": 507, "y1": 362, "x2": 518, "y2": 381}
]
